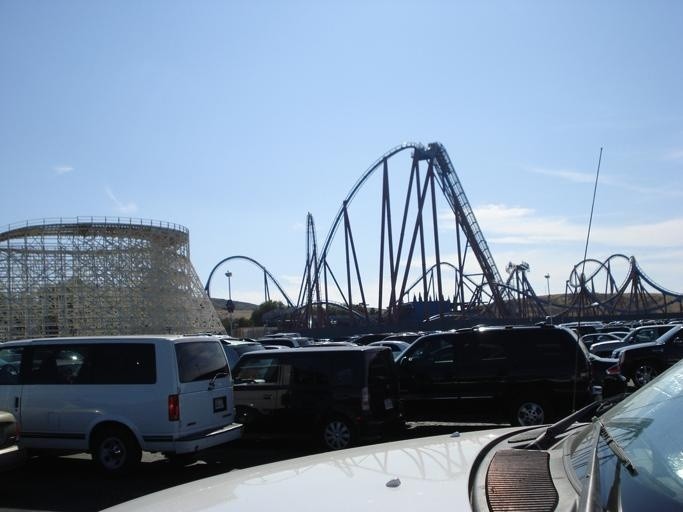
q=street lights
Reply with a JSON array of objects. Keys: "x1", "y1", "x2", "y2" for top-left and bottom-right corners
[
  {"x1": 544, "y1": 274, "x2": 552, "y2": 326},
  {"x1": 225, "y1": 270, "x2": 232, "y2": 336}
]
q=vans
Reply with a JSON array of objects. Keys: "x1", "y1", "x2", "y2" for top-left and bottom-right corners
[{"x1": 0, "y1": 334, "x2": 242, "y2": 476}]
